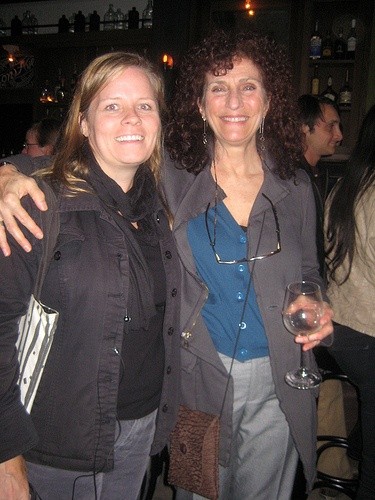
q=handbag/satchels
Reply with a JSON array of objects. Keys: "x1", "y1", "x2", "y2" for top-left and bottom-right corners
[
  {"x1": 167, "y1": 410, "x2": 221, "y2": 500},
  {"x1": 14, "y1": 176, "x2": 60, "y2": 416}
]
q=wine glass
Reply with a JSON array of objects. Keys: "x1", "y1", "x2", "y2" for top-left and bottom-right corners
[{"x1": 282, "y1": 280, "x2": 325, "y2": 390}]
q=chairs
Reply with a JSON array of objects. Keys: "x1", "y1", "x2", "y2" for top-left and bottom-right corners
[{"x1": 313, "y1": 344, "x2": 361, "y2": 500}]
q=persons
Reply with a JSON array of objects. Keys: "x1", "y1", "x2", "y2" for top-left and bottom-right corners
[{"x1": 0, "y1": 27, "x2": 375, "y2": 500}]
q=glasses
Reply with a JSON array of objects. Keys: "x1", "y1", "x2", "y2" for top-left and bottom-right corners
[
  {"x1": 205, "y1": 193, "x2": 282, "y2": 265},
  {"x1": 23, "y1": 142, "x2": 43, "y2": 150}
]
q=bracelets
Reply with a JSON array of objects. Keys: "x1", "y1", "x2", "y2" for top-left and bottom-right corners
[{"x1": 0, "y1": 161, "x2": 10, "y2": 167}]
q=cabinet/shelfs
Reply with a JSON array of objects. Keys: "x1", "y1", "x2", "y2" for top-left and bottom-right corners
[
  {"x1": 299, "y1": 0, "x2": 366, "y2": 155},
  {"x1": 1, "y1": 6, "x2": 157, "y2": 104}
]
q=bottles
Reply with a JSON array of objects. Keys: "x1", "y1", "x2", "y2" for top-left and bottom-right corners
[
  {"x1": 103, "y1": 4, "x2": 115, "y2": 32},
  {"x1": 74, "y1": 11, "x2": 85, "y2": 32},
  {"x1": 142, "y1": 0, "x2": 153, "y2": 32},
  {"x1": 114, "y1": 8, "x2": 125, "y2": 30},
  {"x1": 89, "y1": 10, "x2": 100, "y2": 32},
  {"x1": 58, "y1": 14, "x2": 69, "y2": 33},
  {"x1": 310, "y1": 65, "x2": 320, "y2": 96},
  {"x1": 347, "y1": 17, "x2": 355, "y2": 60},
  {"x1": 339, "y1": 68, "x2": 351, "y2": 107},
  {"x1": 22, "y1": 10, "x2": 33, "y2": 35},
  {"x1": 128, "y1": 7, "x2": 139, "y2": 31},
  {"x1": 40, "y1": 63, "x2": 80, "y2": 104},
  {"x1": 320, "y1": 74, "x2": 338, "y2": 104},
  {"x1": 309, "y1": 20, "x2": 321, "y2": 60},
  {"x1": 11, "y1": 15, "x2": 21, "y2": 36},
  {"x1": 334, "y1": 23, "x2": 346, "y2": 61},
  {"x1": 321, "y1": 23, "x2": 334, "y2": 60}
]
